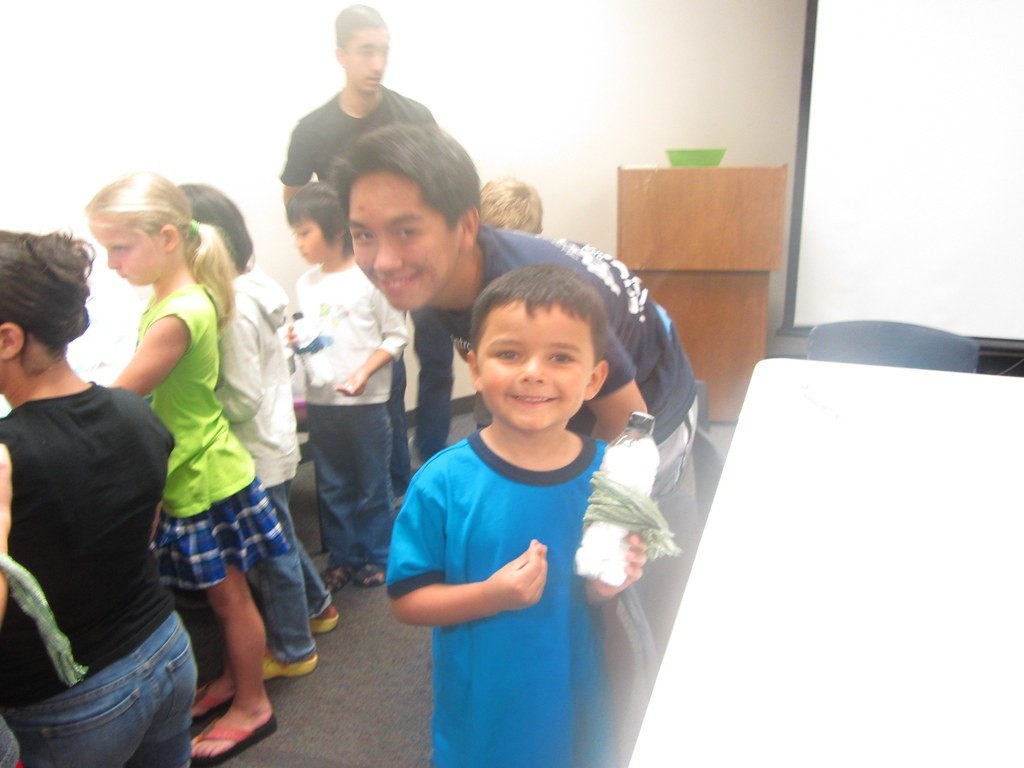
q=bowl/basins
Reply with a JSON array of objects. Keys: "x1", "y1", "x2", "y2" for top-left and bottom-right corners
[{"x1": 666, "y1": 147, "x2": 727, "y2": 168}]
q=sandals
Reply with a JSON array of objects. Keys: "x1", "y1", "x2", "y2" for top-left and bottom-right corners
[
  {"x1": 315, "y1": 563, "x2": 385, "y2": 594},
  {"x1": 260, "y1": 614, "x2": 339, "y2": 682}
]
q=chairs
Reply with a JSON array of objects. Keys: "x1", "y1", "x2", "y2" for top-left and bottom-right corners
[{"x1": 808, "y1": 320, "x2": 981, "y2": 373}]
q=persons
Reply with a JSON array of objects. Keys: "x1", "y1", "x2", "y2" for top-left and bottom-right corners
[
  {"x1": 477, "y1": 171, "x2": 544, "y2": 241},
  {"x1": 1, "y1": 226, "x2": 196, "y2": 767},
  {"x1": 287, "y1": 183, "x2": 413, "y2": 592},
  {"x1": 80, "y1": 174, "x2": 278, "y2": 763},
  {"x1": 385, "y1": 267, "x2": 650, "y2": 766},
  {"x1": 283, "y1": 8, "x2": 455, "y2": 508},
  {"x1": 175, "y1": 180, "x2": 341, "y2": 680},
  {"x1": 332, "y1": 125, "x2": 699, "y2": 763}
]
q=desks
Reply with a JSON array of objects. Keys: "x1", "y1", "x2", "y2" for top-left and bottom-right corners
[{"x1": 630, "y1": 357, "x2": 1024, "y2": 768}]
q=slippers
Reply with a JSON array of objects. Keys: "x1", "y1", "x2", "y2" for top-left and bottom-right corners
[{"x1": 189, "y1": 678, "x2": 278, "y2": 768}]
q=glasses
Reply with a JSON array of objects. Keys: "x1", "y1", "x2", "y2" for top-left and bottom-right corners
[{"x1": 345, "y1": 45, "x2": 388, "y2": 60}]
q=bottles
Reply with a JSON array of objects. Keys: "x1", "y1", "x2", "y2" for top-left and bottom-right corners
[
  {"x1": 574, "y1": 411, "x2": 659, "y2": 588},
  {"x1": 294, "y1": 311, "x2": 335, "y2": 387}
]
